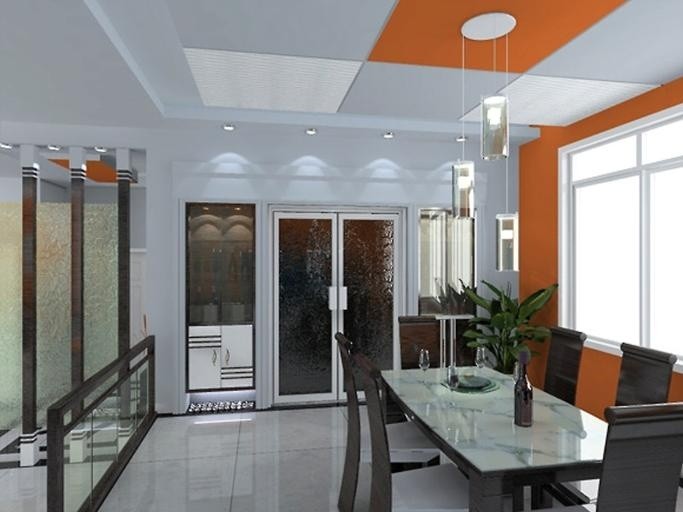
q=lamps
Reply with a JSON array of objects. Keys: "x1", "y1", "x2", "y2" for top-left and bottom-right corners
[{"x1": 450, "y1": 12, "x2": 523, "y2": 275}]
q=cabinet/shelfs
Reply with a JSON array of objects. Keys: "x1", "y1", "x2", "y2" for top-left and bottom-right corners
[{"x1": 187, "y1": 324, "x2": 256, "y2": 392}]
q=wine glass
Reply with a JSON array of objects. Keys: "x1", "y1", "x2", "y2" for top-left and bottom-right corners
[{"x1": 417, "y1": 347, "x2": 431, "y2": 383}]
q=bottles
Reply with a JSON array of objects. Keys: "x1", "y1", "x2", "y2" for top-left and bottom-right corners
[{"x1": 513, "y1": 350, "x2": 534, "y2": 427}]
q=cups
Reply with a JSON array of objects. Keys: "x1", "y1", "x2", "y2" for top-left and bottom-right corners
[
  {"x1": 474, "y1": 347, "x2": 486, "y2": 368},
  {"x1": 447, "y1": 366, "x2": 458, "y2": 389}
]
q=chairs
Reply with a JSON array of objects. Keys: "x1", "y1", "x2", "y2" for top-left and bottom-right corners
[
  {"x1": 540, "y1": 323, "x2": 682, "y2": 511},
  {"x1": 330, "y1": 333, "x2": 470, "y2": 512}
]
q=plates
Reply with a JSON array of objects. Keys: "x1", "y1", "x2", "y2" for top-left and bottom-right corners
[{"x1": 456, "y1": 374, "x2": 491, "y2": 389}]
